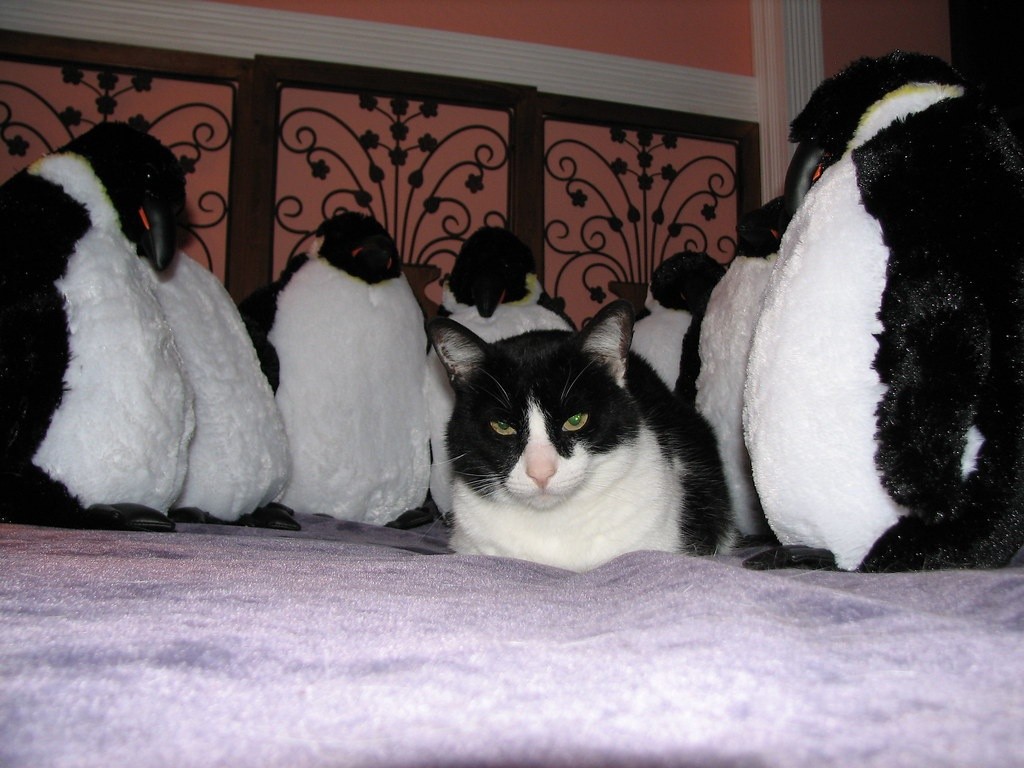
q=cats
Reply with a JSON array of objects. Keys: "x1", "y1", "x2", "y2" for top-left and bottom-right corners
[{"x1": 414, "y1": 298, "x2": 713, "y2": 574}]
